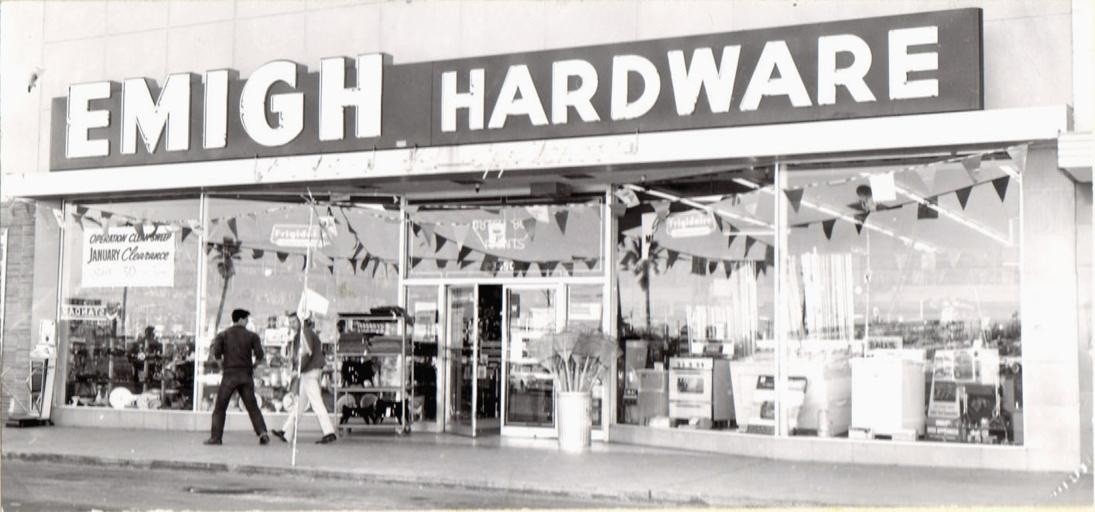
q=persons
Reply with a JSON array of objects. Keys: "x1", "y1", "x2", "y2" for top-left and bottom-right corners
[
  {"x1": 271, "y1": 312, "x2": 336, "y2": 443},
  {"x1": 203, "y1": 309, "x2": 270, "y2": 445}
]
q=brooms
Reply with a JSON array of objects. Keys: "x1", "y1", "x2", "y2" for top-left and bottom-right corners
[{"x1": 525, "y1": 327, "x2": 625, "y2": 392}]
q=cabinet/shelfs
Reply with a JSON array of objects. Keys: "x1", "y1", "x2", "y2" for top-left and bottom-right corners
[{"x1": 334, "y1": 311, "x2": 413, "y2": 434}]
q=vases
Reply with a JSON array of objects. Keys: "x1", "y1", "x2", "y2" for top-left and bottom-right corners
[{"x1": 557, "y1": 391, "x2": 592, "y2": 454}]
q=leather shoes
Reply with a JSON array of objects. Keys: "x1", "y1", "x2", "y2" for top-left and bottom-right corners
[
  {"x1": 260, "y1": 434, "x2": 270, "y2": 444},
  {"x1": 271, "y1": 428, "x2": 287, "y2": 443},
  {"x1": 203, "y1": 437, "x2": 223, "y2": 446},
  {"x1": 315, "y1": 433, "x2": 337, "y2": 445}
]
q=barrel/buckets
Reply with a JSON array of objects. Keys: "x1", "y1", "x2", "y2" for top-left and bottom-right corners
[{"x1": 556, "y1": 390, "x2": 594, "y2": 449}]
